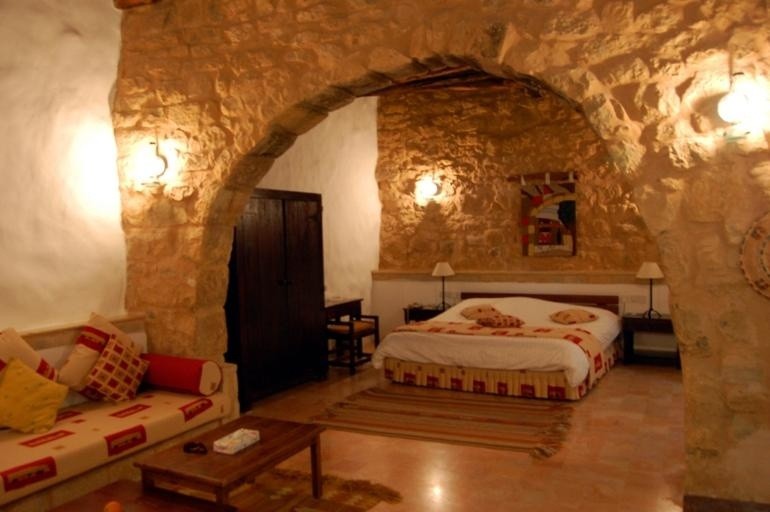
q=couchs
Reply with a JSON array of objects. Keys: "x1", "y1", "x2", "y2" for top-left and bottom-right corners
[{"x1": 1, "y1": 314, "x2": 241, "y2": 511}]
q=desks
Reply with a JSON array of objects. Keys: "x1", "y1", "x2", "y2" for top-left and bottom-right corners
[
  {"x1": 622, "y1": 311, "x2": 680, "y2": 369},
  {"x1": 324, "y1": 295, "x2": 364, "y2": 364},
  {"x1": 402, "y1": 303, "x2": 445, "y2": 323}
]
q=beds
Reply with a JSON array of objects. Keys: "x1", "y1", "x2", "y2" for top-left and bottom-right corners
[{"x1": 373, "y1": 292, "x2": 625, "y2": 400}]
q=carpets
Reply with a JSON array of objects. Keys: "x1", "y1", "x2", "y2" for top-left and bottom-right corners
[
  {"x1": 308, "y1": 384, "x2": 574, "y2": 458},
  {"x1": 228, "y1": 466, "x2": 403, "y2": 511}
]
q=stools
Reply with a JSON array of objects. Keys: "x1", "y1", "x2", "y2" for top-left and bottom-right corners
[{"x1": 327, "y1": 313, "x2": 379, "y2": 375}]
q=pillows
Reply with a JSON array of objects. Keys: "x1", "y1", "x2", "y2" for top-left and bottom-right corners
[
  {"x1": 460, "y1": 303, "x2": 500, "y2": 319},
  {"x1": 548, "y1": 308, "x2": 597, "y2": 326},
  {"x1": 476, "y1": 313, "x2": 525, "y2": 329},
  {"x1": 1, "y1": 310, "x2": 223, "y2": 437}
]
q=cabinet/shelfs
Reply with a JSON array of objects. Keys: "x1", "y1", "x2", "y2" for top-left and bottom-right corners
[{"x1": 231, "y1": 187, "x2": 329, "y2": 415}]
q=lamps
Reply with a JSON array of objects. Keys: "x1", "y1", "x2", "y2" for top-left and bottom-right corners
[
  {"x1": 636, "y1": 263, "x2": 664, "y2": 317},
  {"x1": 432, "y1": 263, "x2": 455, "y2": 308}
]
